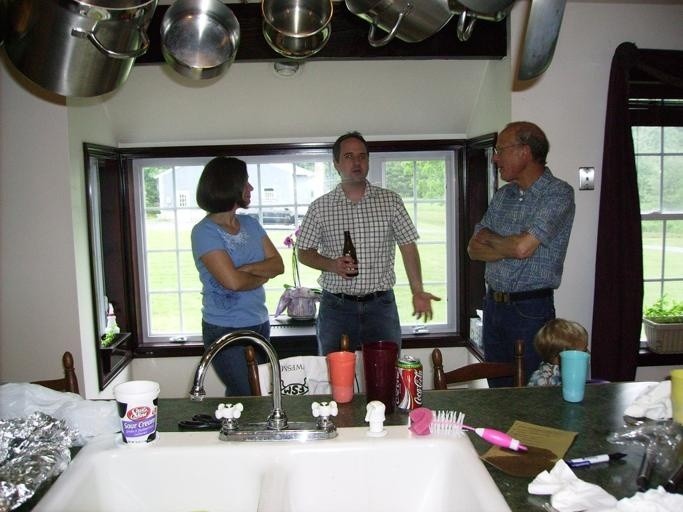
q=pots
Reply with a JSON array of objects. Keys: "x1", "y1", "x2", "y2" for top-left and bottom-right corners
[
  {"x1": 515, "y1": 0, "x2": 566, "y2": 82},
  {"x1": 261, "y1": 0, "x2": 333, "y2": 62},
  {"x1": 443, "y1": 0, "x2": 518, "y2": 43},
  {"x1": 160, "y1": 0, "x2": 241, "y2": 80},
  {"x1": 0, "y1": 0, "x2": 156, "y2": 100},
  {"x1": 344, "y1": 0, "x2": 455, "y2": 50}
]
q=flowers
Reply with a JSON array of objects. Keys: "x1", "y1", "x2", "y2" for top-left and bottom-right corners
[{"x1": 283, "y1": 224, "x2": 320, "y2": 294}]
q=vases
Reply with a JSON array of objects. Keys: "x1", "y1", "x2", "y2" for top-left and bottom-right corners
[{"x1": 274, "y1": 286, "x2": 320, "y2": 322}]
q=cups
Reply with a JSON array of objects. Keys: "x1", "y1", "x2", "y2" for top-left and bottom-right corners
[
  {"x1": 112, "y1": 380, "x2": 160, "y2": 444},
  {"x1": 326, "y1": 350, "x2": 357, "y2": 405},
  {"x1": 359, "y1": 340, "x2": 401, "y2": 413},
  {"x1": 670, "y1": 369, "x2": 682, "y2": 425},
  {"x1": 558, "y1": 349, "x2": 590, "y2": 403}
]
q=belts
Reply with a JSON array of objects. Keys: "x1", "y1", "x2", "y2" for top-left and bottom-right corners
[
  {"x1": 488, "y1": 287, "x2": 554, "y2": 302},
  {"x1": 335, "y1": 291, "x2": 385, "y2": 302}
]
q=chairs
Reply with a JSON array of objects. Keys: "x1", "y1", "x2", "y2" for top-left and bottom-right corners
[
  {"x1": 245, "y1": 334, "x2": 349, "y2": 395},
  {"x1": 0, "y1": 351, "x2": 79, "y2": 393},
  {"x1": 432, "y1": 337, "x2": 528, "y2": 390}
]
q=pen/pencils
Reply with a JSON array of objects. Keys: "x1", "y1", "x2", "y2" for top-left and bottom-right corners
[{"x1": 565, "y1": 452, "x2": 628, "y2": 467}]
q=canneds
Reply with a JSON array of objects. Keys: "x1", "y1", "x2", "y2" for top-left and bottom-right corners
[{"x1": 396, "y1": 354, "x2": 423, "y2": 414}]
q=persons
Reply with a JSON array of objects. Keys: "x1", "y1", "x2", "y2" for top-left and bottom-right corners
[
  {"x1": 191, "y1": 156, "x2": 285, "y2": 397},
  {"x1": 525, "y1": 318, "x2": 591, "y2": 387},
  {"x1": 468, "y1": 120, "x2": 575, "y2": 389},
  {"x1": 297, "y1": 132, "x2": 442, "y2": 360}
]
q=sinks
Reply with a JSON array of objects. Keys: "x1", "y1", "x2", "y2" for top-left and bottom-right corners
[
  {"x1": 256, "y1": 426, "x2": 513, "y2": 512},
  {"x1": 29, "y1": 432, "x2": 259, "y2": 512}
]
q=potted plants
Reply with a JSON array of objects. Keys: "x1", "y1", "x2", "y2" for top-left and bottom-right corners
[{"x1": 642, "y1": 293, "x2": 683, "y2": 355}]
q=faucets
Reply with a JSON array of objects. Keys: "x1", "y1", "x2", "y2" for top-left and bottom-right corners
[{"x1": 190, "y1": 328, "x2": 289, "y2": 431}]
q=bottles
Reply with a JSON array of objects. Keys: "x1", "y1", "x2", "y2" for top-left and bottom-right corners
[{"x1": 342, "y1": 231, "x2": 359, "y2": 277}]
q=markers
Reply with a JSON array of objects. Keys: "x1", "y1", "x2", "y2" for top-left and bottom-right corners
[
  {"x1": 663, "y1": 465, "x2": 683, "y2": 492},
  {"x1": 635, "y1": 447, "x2": 655, "y2": 491}
]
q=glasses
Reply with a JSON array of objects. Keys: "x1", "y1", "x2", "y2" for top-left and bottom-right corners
[{"x1": 494, "y1": 143, "x2": 525, "y2": 156}]
